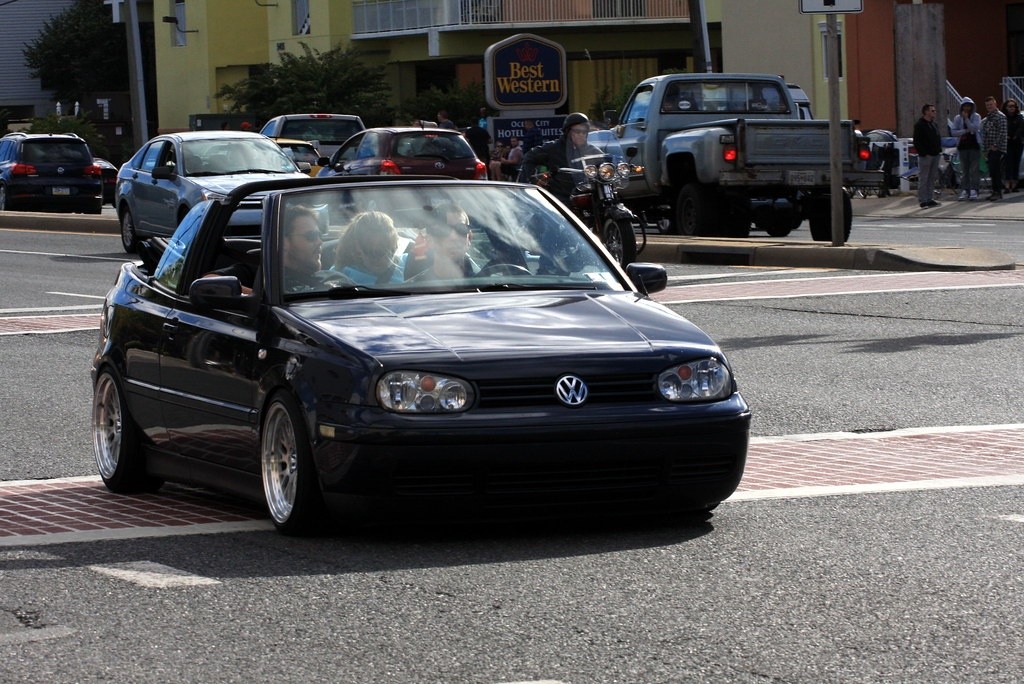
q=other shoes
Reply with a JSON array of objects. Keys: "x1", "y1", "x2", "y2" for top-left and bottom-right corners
[
  {"x1": 969, "y1": 189, "x2": 978, "y2": 200},
  {"x1": 1012, "y1": 187, "x2": 1020, "y2": 192},
  {"x1": 920, "y1": 199, "x2": 940, "y2": 208},
  {"x1": 985, "y1": 189, "x2": 1002, "y2": 201},
  {"x1": 957, "y1": 189, "x2": 969, "y2": 201},
  {"x1": 1003, "y1": 187, "x2": 1010, "y2": 193}
]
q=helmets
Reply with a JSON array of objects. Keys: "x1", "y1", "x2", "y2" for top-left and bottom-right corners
[{"x1": 562, "y1": 112, "x2": 591, "y2": 133}]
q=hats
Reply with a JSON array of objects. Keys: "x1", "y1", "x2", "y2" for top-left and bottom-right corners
[{"x1": 240, "y1": 121, "x2": 252, "y2": 128}]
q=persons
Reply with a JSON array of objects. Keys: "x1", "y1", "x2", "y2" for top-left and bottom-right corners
[
  {"x1": 202, "y1": 205, "x2": 498, "y2": 294},
  {"x1": 912, "y1": 96, "x2": 1024, "y2": 208},
  {"x1": 414, "y1": 105, "x2": 612, "y2": 219}
]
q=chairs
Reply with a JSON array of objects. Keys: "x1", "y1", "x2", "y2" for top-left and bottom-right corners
[{"x1": 212, "y1": 238, "x2": 339, "y2": 271}]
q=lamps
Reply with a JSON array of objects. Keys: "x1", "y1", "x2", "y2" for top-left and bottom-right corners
[{"x1": 162, "y1": 16, "x2": 199, "y2": 32}]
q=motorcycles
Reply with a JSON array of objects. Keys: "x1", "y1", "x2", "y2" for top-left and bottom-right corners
[{"x1": 531, "y1": 131, "x2": 647, "y2": 270}]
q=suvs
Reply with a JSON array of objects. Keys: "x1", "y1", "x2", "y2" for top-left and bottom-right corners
[{"x1": 0, "y1": 131, "x2": 104, "y2": 214}]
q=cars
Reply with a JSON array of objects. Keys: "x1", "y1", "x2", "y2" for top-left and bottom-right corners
[
  {"x1": 866, "y1": 129, "x2": 919, "y2": 172},
  {"x1": 90, "y1": 178, "x2": 751, "y2": 535},
  {"x1": 113, "y1": 131, "x2": 312, "y2": 252},
  {"x1": 315, "y1": 125, "x2": 492, "y2": 224},
  {"x1": 92, "y1": 156, "x2": 120, "y2": 207}
]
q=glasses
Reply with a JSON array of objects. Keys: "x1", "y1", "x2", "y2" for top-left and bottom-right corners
[
  {"x1": 1007, "y1": 104, "x2": 1016, "y2": 108},
  {"x1": 570, "y1": 128, "x2": 588, "y2": 134},
  {"x1": 430, "y1": 222, "x2": 473, "y2": 238},
  {"x1": 287, "y1": 229, "x2": 324, "y2": 242}
]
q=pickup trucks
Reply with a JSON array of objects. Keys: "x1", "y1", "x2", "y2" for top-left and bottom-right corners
[
  {"x1": 586, "y1": 72, "x2": 870, "y2": 244},
  {"x1": 260, "y1": 114, "x2": 367, "y2": 158}
]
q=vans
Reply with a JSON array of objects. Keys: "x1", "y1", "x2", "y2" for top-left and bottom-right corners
[{"x1": 702, "y1": 85, "x2": 814, "y2": 121}]
